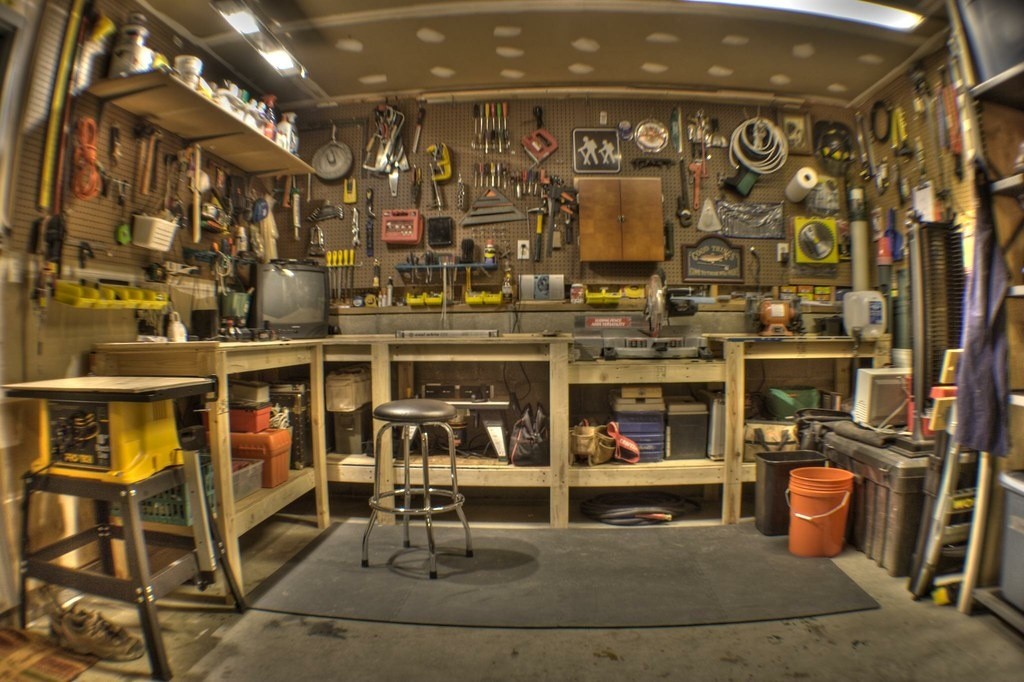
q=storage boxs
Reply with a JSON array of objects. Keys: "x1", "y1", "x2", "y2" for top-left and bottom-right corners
[
  {"x1": 232, "y1": 457, "x2": 265, "y2": 501},
  {"x1": 204, "y1": 427, "x2": 292, "y2": 489},
  {"x1": 229, "y1": 406, "x2": 271, "y2": 433},
  {"x1": 822, "y1": 431, "x2": 979, "y2": 578},
  {"x1": 997, "y1": 466, "x2": 1024, "y2": 610},
  {"x1": 325, "y1": 365, "x2": 371, "y2": 413},
  {"x1": 109, "y1": 451, "x2": 217, "y2": 527},
  {"x1": 610, "y1": 410, "x2": 664, "y2": 463},
  {"x1": 664, "y1": 394, "x2": 710, "y2": 460}
]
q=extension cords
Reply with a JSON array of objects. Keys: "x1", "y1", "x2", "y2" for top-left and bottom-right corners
[{"x1": 165, "y1": 260, "x2": 200, "y2": 276}]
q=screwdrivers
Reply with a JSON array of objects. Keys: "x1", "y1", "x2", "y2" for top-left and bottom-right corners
[
  {"x1": 406, "y1": 251, "x2": 434, "y2": 285},
  {"x1": 469, "y1": 101, "x2": 511, "y2": 155},
  {"x1": 469, "y1": 160, "x2": 538, "y2": 199}
]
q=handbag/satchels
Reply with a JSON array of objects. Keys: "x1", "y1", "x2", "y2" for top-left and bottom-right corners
[
  {"x1": 796, "y1": 408, "x2": 853, "y2": 453},
  {"x1": 509, "y1": 402, "x2": 550, "y2": 467},
  {"x1": 743, "y1": 418, "x2": 800, "y2": 461}
]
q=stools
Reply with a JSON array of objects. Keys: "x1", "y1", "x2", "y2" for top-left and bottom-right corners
[{"x1": 361, "y1": 398, "x2": 475, "y2": 579}]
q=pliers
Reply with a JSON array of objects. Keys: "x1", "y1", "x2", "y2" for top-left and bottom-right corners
[{"x1": 410, "y1": 169, "x2": 421, "y2": 207}]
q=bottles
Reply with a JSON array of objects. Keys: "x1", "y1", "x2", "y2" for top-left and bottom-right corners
[
  {"x1": 843, "y1": 289, "x2": 887, "y2": 342},
  {"x1": 168, "y1": 320, "x2": 187, "y2": 342},
  {"x1": 210, "y1": 78, "x2": 300, "y2": 158},
  {"x1": 484, "y1": 239, "x2": 495, "y2": 264},
  {"x1": 570, "y1": 284, "x2": 585, "y2": 303}
]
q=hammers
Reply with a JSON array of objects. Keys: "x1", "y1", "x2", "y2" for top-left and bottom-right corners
[
  {"x1": 528, "y1": 207, "x2": 545, "y2": 262},
  {"x1": 133, "y1": 120, "x2": 166, "y2": 191}
]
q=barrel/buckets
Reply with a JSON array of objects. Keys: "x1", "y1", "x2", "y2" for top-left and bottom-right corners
[
  {"x1": 892, "y1": 348, "x2": 912, "y2": 369},
  {"x1": 785, "y1": 467, "x2": 854, "y2": 557}
]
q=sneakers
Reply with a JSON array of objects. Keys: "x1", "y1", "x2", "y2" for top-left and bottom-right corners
[{"x1": 49, "y1": 603, "x2": 146, "y2": 661}]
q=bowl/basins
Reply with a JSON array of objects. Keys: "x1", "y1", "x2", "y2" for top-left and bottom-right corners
[{"x1": 108, "y1": 12, "x2": 203, "y2": 91}]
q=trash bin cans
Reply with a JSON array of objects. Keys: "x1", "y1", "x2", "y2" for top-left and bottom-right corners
[
  {"x1": 754, "y1": 448, "x2": 825, "y2": 535},
  {"x1": 997, "y1": 469, "x2": 1024, "y2": 614}
]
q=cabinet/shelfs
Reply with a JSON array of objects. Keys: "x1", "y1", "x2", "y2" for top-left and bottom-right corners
[
  {"x1": 87, "y1": 328, "x2": 894, "y2": 605},
  {"x1": 572, "y1": 176, "x2": 666, "y2": 261},
  {"x1": 945, "y1": 0, "x2": 1024, "y2": 635}
]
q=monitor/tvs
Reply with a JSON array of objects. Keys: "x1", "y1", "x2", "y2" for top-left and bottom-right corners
[{"x1": 249, "y1": 262, "x2": 330, "y2": 339}]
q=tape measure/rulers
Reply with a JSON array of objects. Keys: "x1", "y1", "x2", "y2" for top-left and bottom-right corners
[
  {"x1": 252, "y1": 199, "x2": 269, "y2": 220},
  {"x1": 463, "y1": 187, "x2": 528, "y2": 225}
]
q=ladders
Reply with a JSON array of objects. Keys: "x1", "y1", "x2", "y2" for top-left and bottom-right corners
[{"x1": 908, "y1": 400, "x2": 984, "y2": 600}]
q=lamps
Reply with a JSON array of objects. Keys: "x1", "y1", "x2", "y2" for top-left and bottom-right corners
[{"x1": 209, "y1": 0, "x2": 310, "y2": 78}]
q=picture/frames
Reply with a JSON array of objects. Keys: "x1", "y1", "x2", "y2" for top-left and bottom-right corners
[
  {"x1": 775, "y1": 108, "x2": 814, "y2": 156},
  {"x1": 680, "y1": 233, "x2": 746, "y2": 284}
]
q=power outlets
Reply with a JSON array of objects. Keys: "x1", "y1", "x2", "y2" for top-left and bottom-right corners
[
  {"x1": 517, "y1": 240, "x2": 530, "y2": 260},
  {"x1": 777, "y1": 243, "x2": 790, "y2": 262}
]
